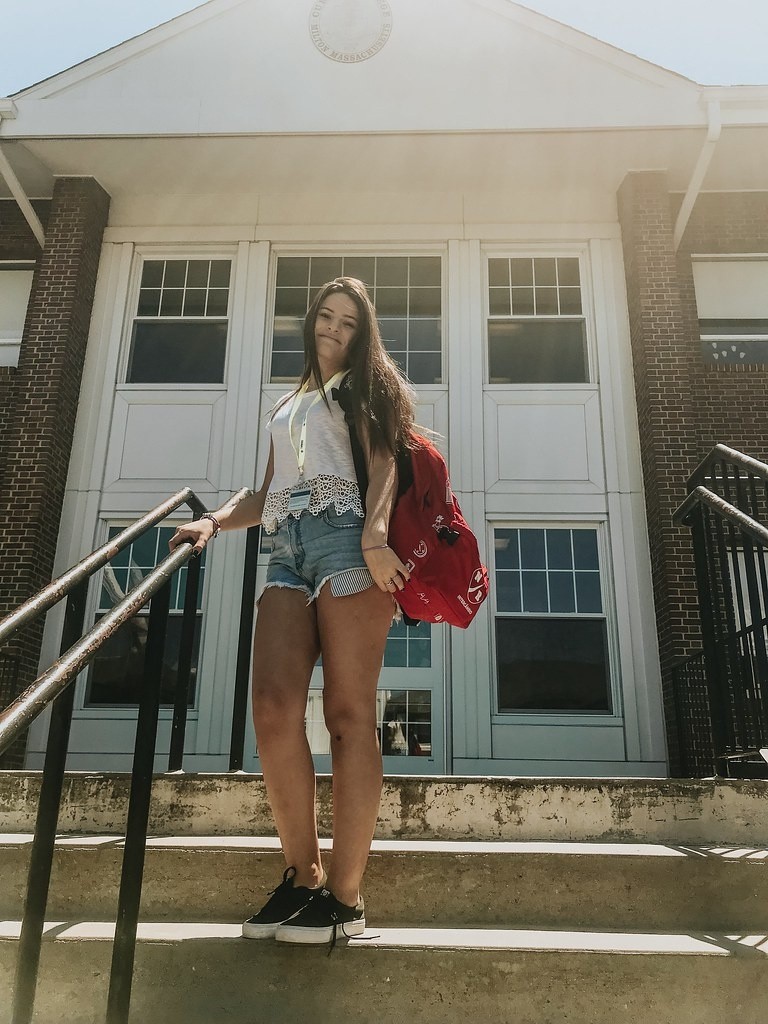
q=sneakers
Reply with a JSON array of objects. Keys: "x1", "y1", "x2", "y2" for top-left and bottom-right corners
[
  {"x1": 242, "y1": 866, "x2": 328, "y2": 939},
  {"x1": 275, "y1": 888, "x2": 380, "y2": 957}
]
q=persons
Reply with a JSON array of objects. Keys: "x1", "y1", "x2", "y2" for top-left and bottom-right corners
[
  {"x1": 169, "y1": 276, "x2": 443, "y2": 957},
  {"x1": 384, "y1": 705, "x2": 418, "y2": 756}
]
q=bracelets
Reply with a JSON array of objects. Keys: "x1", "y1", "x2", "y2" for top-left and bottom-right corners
[
  {"x1": 362, "y1": 545, "x2": 388, "y2": 552},
  {"x1": 200, "y1": 513, "x2": 221, "y2": 538}
]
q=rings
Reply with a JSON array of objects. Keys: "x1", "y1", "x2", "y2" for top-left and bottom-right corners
[
  {"x1": 392, "y1": 574, "x2": 398, "y2": 579},
  {"x1": 386, "y1": 581, "x2": 393, "y2": 586}
]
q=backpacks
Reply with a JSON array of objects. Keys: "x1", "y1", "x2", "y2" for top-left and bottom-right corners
[{"x1": 390, "y1": 424, "x2": 490, "y2": 628}]
